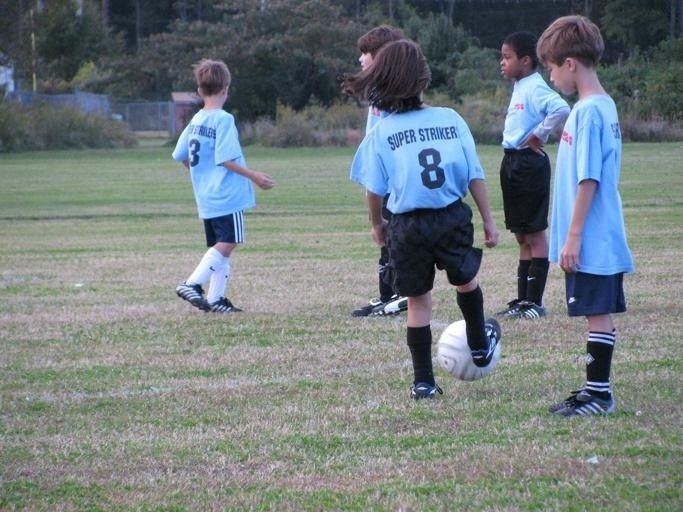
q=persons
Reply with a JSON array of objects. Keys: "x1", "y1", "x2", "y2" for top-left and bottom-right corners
[
  {"x1": 493, "y1": 31, "x2": 571, "y2": 319},
  {"x1": 535, "y1": 15, "x2": 634, "y2": 417},
  {"x1": 172, "y1": 59, "x2": 275, "y2": 313},
  {"x1": 337, "y1": 41, "x2": 501, "y2": 400},
  {"x1": 351, "y1": 24, "x2": 407, "y2": 317}
]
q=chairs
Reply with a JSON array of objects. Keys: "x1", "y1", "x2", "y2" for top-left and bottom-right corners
[
  {"x1": 495, "y1": 297, "x2": 546, "y2": 323},
  {"x1": 552, "y1": 389, "x2": 611, "y2": 418},
  {"x1": 206, "y1": 299, "x2": 240, "y2": 314},
  {"x1": 410, "y1": 382, "x2": 442, "y2": 400},
  {"x1": 471, "y1": 316, "x2": 503, "y2": 367},
  {"x1": 178, "y1": 280, "x2": 209, "y2": 312},
  {"x1": 354, "y1": 297, "x2": 409, "y2": 317}
]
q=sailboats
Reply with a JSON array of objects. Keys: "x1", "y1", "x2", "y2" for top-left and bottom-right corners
[{"x1": 436, "y1": 318, "x2": 504, "y2": 383}]
